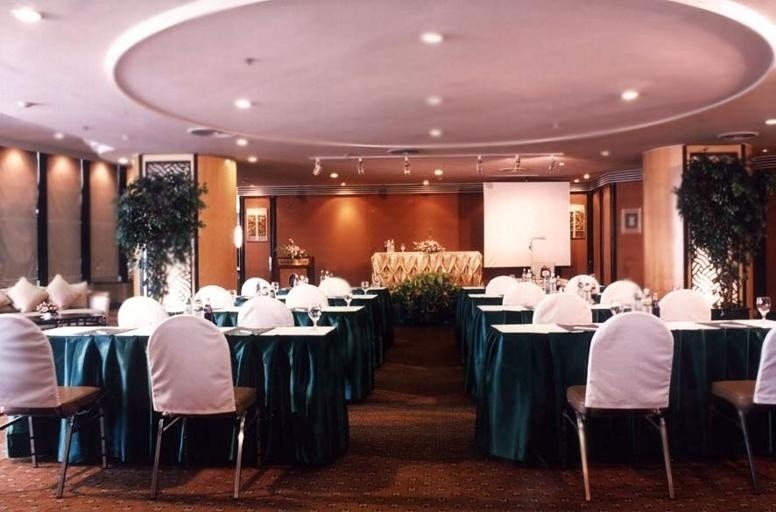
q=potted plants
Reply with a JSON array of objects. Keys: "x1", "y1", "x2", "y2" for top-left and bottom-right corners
[{"x1": 672, "y1": 144, "x2": 776, "y2": 322}]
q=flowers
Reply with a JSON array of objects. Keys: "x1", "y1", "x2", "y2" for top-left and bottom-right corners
[{"x1": 413, "y1": 239, "x2": 445, "y2": 253}]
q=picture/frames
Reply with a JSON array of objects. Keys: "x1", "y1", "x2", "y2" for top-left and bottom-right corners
[{"x1": 620, "y1": 207, "x2": 642, "y2": 235}]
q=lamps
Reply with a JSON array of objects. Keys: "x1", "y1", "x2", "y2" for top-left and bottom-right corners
[{"x1": 306, "y1": 151, "x2": 566, "y2": 177}]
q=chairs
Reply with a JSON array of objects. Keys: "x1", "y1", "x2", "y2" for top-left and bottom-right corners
[
  {"x1": 458, "y1": 274, "x2": 776, "y2": 502},
  {"x1": 0, "y1": 274, "x2": 398, "y2": 502}
]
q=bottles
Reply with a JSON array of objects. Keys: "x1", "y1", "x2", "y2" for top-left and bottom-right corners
[
  {"x1": 255, "y1": 270, "x2": 334, "y2": 300},
  {"x1": 521, "y1": 266, "x2": 660, "y2": 319},
  {"x1": 184, "y1": 297, "x2": 213, "y2": 323},
  {"x1": 384, "y1": 239, "x2": 396, "y2": 252}
]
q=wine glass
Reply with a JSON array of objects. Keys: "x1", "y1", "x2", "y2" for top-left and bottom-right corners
[
  {"x1": 344, "y1": 293, "x2": 352, "y2": 308},
  {"x1": 308, "y1": 305, "x2": 322, "y2": 330},
  {"x1": 757, "y1": 297, "x2": 772, "y2": 320},
  {"x1": 361, "y1": 282, "x2": 370, "y2": 296},
  {"x1": 400, "y1": 243, "x2": 406, "y2": 252}
]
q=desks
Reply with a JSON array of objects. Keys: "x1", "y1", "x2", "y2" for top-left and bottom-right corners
[{"x1": 369, "y1": 251, "x2": 484, "y2": 288}]
